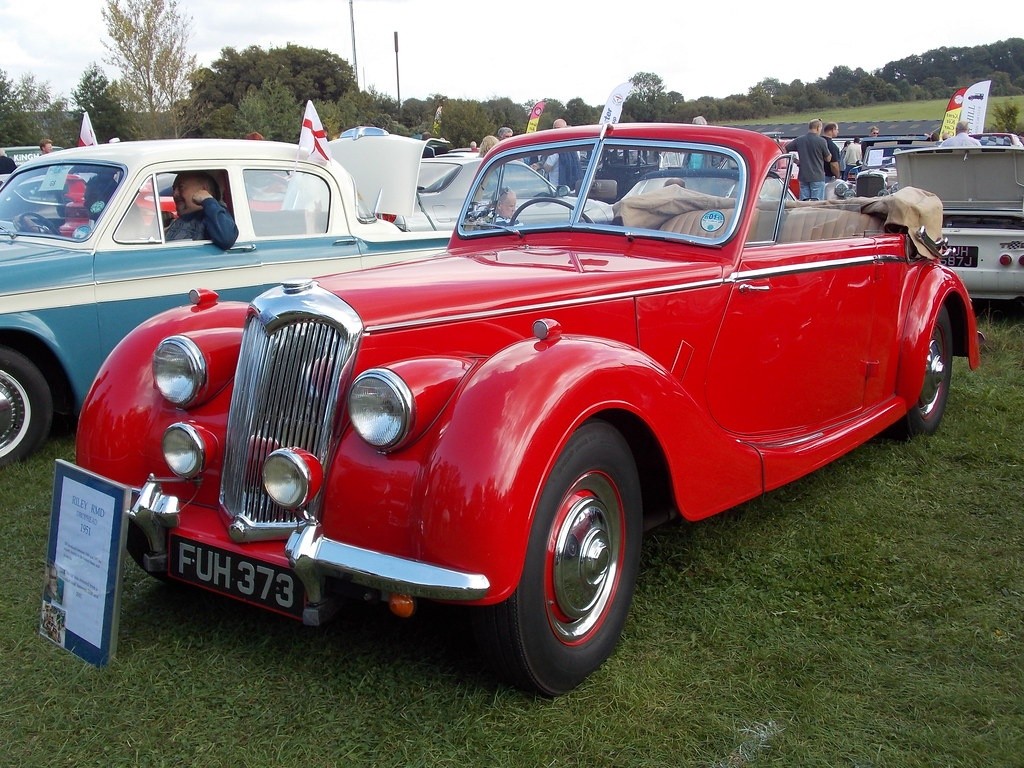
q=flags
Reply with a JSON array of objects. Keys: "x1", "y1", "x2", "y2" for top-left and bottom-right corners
[
  {"x1": 597, "y1": 82, "x2": 634, "y2": 124},
  {"x1": 297, "y1": 99, "x2": 334, "y2": 163},
  {"x1": 76, "y1": 111, "x2": 98, "y2": 149},
  {"x1": 939, "y1": 86, "x2": 967, "y2": 141},
  {"x1": 957, "y1": 79, "x2": 993, "y2": 134},
  {"x1": 527, "y1": 101, "x2": 548, "y2": 135},
  {"x1": 432, "y1": 104, "x2": 444, "y2": 135}
]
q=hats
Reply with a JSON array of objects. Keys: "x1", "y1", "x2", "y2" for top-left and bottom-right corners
[{"x1": 109, "y1": 137, "x2": 120, "y2": 143}]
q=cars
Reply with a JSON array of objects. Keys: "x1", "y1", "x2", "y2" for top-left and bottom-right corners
[
  {"x1": 834, "y1": 132, "x2": 1024, "y2": 302},
  {"x1": 548, "y1": 141, "x2": 934, "y2": 196},
  {"x1": 72, "y1": 122, "x2": 982, "y2": 699},
  {"x1": 0, "y1": 127, "x2": 615, "y2": 461}
]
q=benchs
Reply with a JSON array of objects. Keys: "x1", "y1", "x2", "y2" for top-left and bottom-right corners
[
  {"x1": 252, "y1": 209, "x2": 328, "y2": 237},
  {"x1": 659, "y1": 207, "x2": 887, "y2": 243}
]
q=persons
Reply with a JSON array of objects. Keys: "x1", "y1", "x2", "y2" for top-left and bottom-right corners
[
  {"x1": 422, "y1": 119, "x2": 583, "y2": 230},
  {"x1": 931, "y1": 120, "x2": 982, "y2": 148},
  {"x1": 163, "y1": 171, "x2": 239, "y2": 250},
  {"x1": 660, "y1": 115, "x2": 880, "y2": 201},
  {"x1": 0, "y1": 138, "x2": 53, "y2": 175},
  {"x1": 13, "y1": 172, "x2": 117, "y2": 231}
]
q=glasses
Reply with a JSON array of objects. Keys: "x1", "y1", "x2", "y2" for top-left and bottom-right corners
[
  {"x1": 500, "y1": 186, "x2": 511, "y2": 196},
  {"x1": 818, "y1": 118, "x2": 822, "y2": 127},
  {"x1": 872, "y1": 131, "x2": 878, "y2": 134}
]
